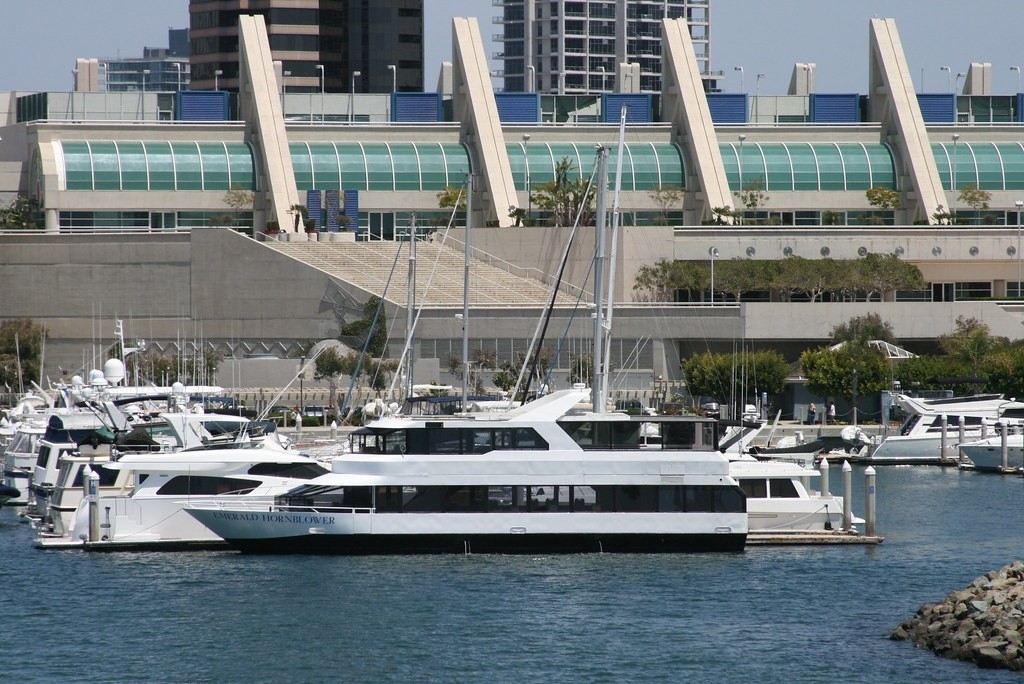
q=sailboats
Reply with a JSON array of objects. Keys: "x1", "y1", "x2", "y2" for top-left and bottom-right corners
[{"x1": 0, "y1": 100, "x2": 1024, "y2": 553}]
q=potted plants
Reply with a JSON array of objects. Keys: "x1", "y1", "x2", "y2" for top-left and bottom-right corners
[
  {"x1": 279, "y1": 230, "x2": 288, "y2": 241},
  {"x1": 319, "y1": 229, "x2": 330, "y2": 242},
  {"x1": 286, "y1": 203, "x2": 308, "y2": 242},
  {"x1": 264, "y1": 222, "x2": 278, "y2": 241},
  {"x1": 332, "y1": 214, "x2": 355, "y2": 242},
  {"x1": 304, "y1": 219, "x2": 317, "y2": 242}
]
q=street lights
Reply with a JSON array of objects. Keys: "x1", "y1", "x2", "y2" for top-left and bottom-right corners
[
  {"x1": 1009, "y1": 65, "x2": 1021, "y2": 92},
  {"x1": 1015, "y1": 200, "x2": 1024, "y2": 300},
  {"x1": 710, "y1": 246, "x2": 719, "y2": 308},
  {"x1": 596, "y1": 66, "x2": 605, "y2": 92},
  {"x1": 314, "y1": 64, "x2": 325, "y2": 122},
  {"x1": 950, "y1": 133, "x2": 961, "y2": 225},
  {"x1": 756, "y1": 72, "x2": 765, "y2": 127},
  {"x1": 526, "y1": 65, "x2": 534, "y2": 93},
  {"x1": 172, "y1": 62, "x2": 181, "y2": 90},
  {"x1": 282, "y1": 71, "x2": 292, "y2": 117},
  {"x1": 941, "y1": 66, "x2": 952, "y2": 93},
  {"x1": 351, "y1": 70, "x2": 361, "y2": 124},
  {"x1": 734, "y1": 65, "x2": 743, "y2": 93},
  {"x1": 213, "y1": 69, "x2": 223, "y2": 92},
  {"x1": 955, "y1": 72, "x2": 966, "y2": 94},
  {"x1": 141, "y1": 69, "x2": 151, "y2": 124}
]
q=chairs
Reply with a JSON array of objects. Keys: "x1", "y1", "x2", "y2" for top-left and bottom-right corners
[{"x1": 530, "y1": 498, "x2": 585, "y2": 511}]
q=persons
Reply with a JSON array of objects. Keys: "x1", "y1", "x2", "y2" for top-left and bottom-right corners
[
  {"x1": 828, "y1": 400, "x2": 836, "y2": 424},
  {"x1": 425, "y1": 229, "x2": 434, "y2": 243},
  {"x1": 289, "y1": 408, "x2": 297, "y2": 428},
  {"x1": 807, "y1": 401, "x2": 816, "y2": 424}
]
